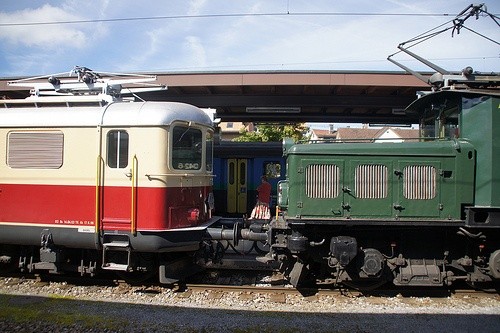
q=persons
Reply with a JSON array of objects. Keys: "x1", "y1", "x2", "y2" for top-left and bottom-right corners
[{"x1": 249, "y1": 175, "x2": 272, "y2": 219}]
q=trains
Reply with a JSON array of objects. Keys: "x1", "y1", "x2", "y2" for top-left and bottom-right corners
[{"x1": 0, "y1": 3, "x2": 500, "y2": 291}]
